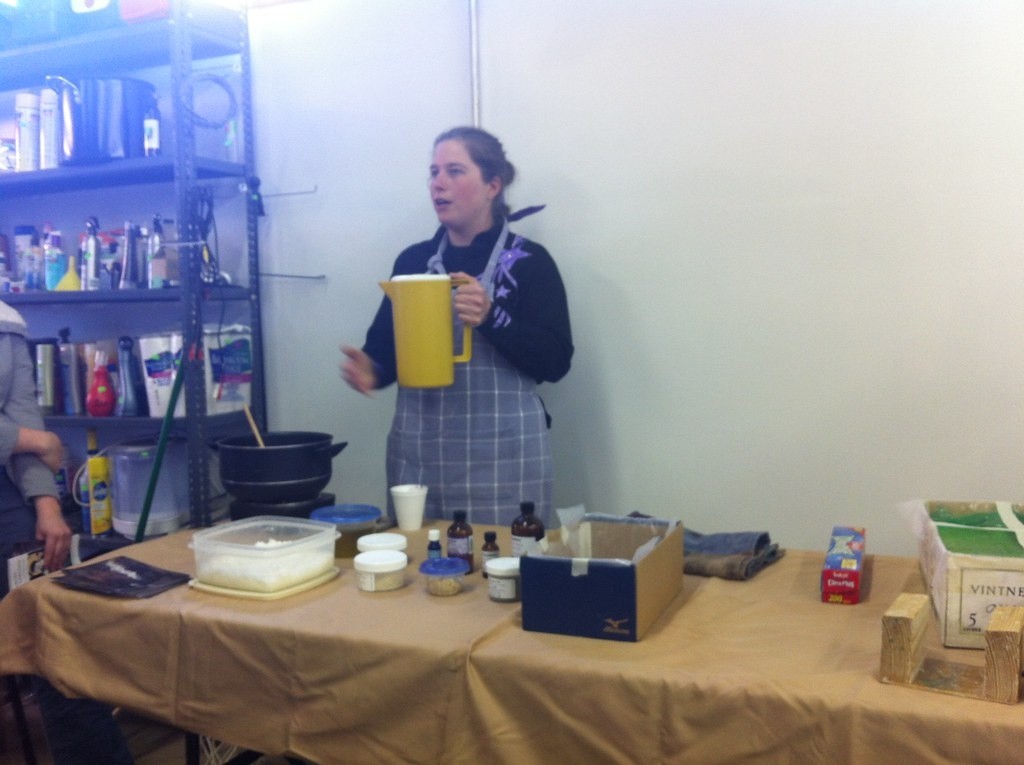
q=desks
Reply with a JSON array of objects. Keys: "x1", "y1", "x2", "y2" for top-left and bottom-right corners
[{"x1": 0, "y1": 510, "x2": 1024, "y2": 765}]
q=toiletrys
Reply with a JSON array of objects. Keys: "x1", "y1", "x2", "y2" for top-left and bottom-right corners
[
  {"x1": 30, "y1": 325, "x2": 115, "y2": 417},
  {"x1": 0, "y1": 213, "x2": 178, "y2": 291},
  {"x1": 113, "y1": 337, "x2": 140, "y2": 417}
]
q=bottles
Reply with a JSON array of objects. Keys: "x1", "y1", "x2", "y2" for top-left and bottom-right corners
[
  {"x1": 142, "y1": 97, "x2": 161, "y2": 157},
  {"x1": 480, "y1": 531, "x2": 500, "y2": 578},
  {"x1": 79, "y1": 427, "x2": 111, "y2": 534},
  {"x1": 34, "y1": 327, "x2": 145, "y2": 417},
  {"x1": 12, "y1": 215, "x2": 179, "y2": 292},
  {"x1": 446, "y1": 510, "x2": 475, "y2": 575},
  {"x1": 510, "y1": 501, "x2": 547, "y2": 557},
  {"x1": 426, "y1": 528, "x2": 443, "y2": 566},
  {"x1": 12, "y1": 87, "x2": 58, "y2": 172}
]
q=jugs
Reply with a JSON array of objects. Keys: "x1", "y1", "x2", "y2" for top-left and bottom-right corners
[{"x1": 376, "y1": 273, "x2": 472, "y2": 389}]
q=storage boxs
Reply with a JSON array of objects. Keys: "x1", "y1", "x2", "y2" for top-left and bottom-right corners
[
  {"x1": 188, "y1": 516, "x2": 342, "y2": 598},
  {"x1": 516, "y1": 513, "x2": 690, "y2": 648},
  {"x1": 819, "y1": 525, "x2": 867, "y2": 604},
  {"x1": 916, "y1": 497, "x2": 1024, "y2": 654}
]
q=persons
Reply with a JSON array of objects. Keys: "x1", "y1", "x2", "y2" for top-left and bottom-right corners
[
  {"x1": 1, "y1": 301, "x2": 74, "y2": 573},
  {"x1": 336, "y1": 127, "x2": 576, "y2": 530}
]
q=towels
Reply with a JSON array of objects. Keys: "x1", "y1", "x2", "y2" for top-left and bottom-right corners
[{"x1": 627, "y1": 510, "x2": 787, "y2": 585}]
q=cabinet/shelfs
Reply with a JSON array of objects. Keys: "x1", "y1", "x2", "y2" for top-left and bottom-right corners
[{"x1": 1, "y1": 1, "x2": 262, "y2": 580}]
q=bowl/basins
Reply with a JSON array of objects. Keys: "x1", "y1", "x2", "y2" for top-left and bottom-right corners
[
  {"x1": 484, "y1": 556, "x2": 523, "y2": 602},
  {"x1": 419, "y1": 557, "x2": 471, "y2": 597},
  {"x1": 353, "y1": 532, "x2": 409, "y2": 593},
  {"x1": 186, "y1": 514, "x2": 342, "y2": 594},
  {"x1": 310, "y1": 503, "x2": 382, "y2": 558}
]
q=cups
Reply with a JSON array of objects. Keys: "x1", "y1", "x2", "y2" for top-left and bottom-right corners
[
  {"x1": 389, "y1": 484, "x2": 429, "y2": 531},
  {"x1": 138, "y1": 334, "x2": 252, "y2": 417}
]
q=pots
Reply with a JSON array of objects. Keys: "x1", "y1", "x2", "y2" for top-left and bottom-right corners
[
  {"x1": 205, "y1": 434, "x2": 349, "y2": 501},
  {"x1": 45, "y1": 75, "x2": 156, "y2": 164}
]
